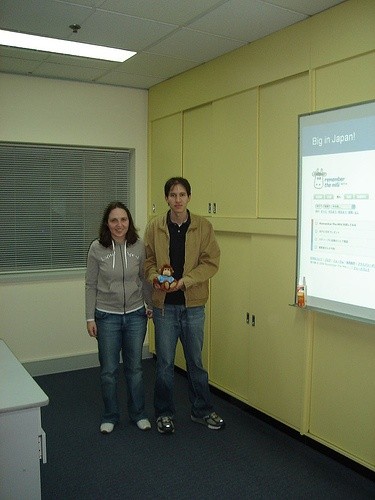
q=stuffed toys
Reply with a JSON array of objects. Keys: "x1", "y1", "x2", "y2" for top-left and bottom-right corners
[{"x1": 153, "y1": 264, "x2": 178, "y2": 290}]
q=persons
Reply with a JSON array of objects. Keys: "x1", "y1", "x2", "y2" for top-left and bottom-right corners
[
  {"x1": 84, "y1": 202, "x2": 154, "y2": 433},
  {"x1": 144, "y1": 178, "x2": 224, "y2": 434}
]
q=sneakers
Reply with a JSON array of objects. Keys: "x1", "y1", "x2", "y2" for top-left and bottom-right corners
[
  {"x1": 157, "y1": 415, "x2": 175, "y2": 434},
  {"x1": 100, "y1": 423, "x2": 115, "y2": 433},
  {"x1": 191, "y1": 411, "x2": 224, "y2": 430},
  {"x1": 137, "y1": 419, "x2": 151, "y2": 430}
]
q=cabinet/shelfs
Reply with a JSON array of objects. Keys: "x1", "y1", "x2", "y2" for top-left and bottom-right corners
[
  {"x1": 147, "y1": 50, "x2": 375, "y2": 474},
  {"x1": 0, "y1": 337, "x2": 49, "y2": 500}
]
